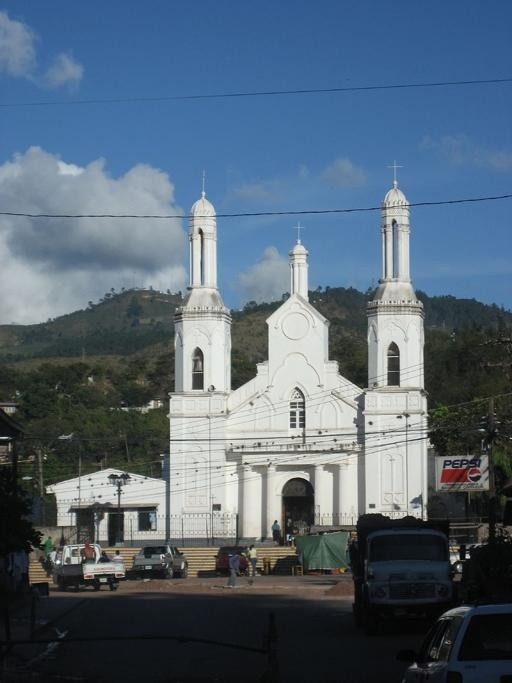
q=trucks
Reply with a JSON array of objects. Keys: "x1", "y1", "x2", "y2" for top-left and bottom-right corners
[
  {"x1": 349, "y1": 513, "x2": 466, "y2": 634},
  {"x1": 53, "y1": 542, "x2": 126, "y2": 591}
]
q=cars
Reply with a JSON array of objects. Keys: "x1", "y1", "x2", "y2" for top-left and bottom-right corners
[
  {"x1": 215, "y1": 546, "x2": 248, "y2": 575},
  {"x1": 400, "y1": 603, "x2": 512, "y2": 683}
]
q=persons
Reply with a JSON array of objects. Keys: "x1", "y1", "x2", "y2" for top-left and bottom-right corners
[
  {"x1": 49, "y1": 547, "x2": 58, "y2": 565},
  {"x1": 272, "y1": 520, "x2": 280, "y2": 544},
  {"x1": 225, "y1": 552, "x2": 241, "y2": 587},
  {"x1": 63, "y1": 541, "x2": 71, "y2": 563},
  {"x1": 346, "y1": 531, "x2": 366, "y2": 600},
  {"x1": 246, "y1": 545, "x2": 257, "y2": 576},
  {"x1": 112, "y1": 551, "x2": 124, "y2": 562},
  {"x1": 43, "y1": 536, "x2": 51, "y2": 555},
  {"x1": 97, "y1": 551, "x2": 111, "y2": 565},
  {"x1": 286, "y1": 518, "x2": 294, "y2": 545},
  {"x1": 79, "y1": 540, "x2": 96, "y2": 564}
]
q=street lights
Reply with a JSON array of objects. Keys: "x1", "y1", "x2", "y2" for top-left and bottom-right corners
[{"x1": 109, "y1": 472, "x2": 130, "y2": 541}]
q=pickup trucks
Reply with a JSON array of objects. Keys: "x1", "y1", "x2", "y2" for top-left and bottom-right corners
[{"x1": 131, "y1": 545, "x2": 188, "y2": 578}]
